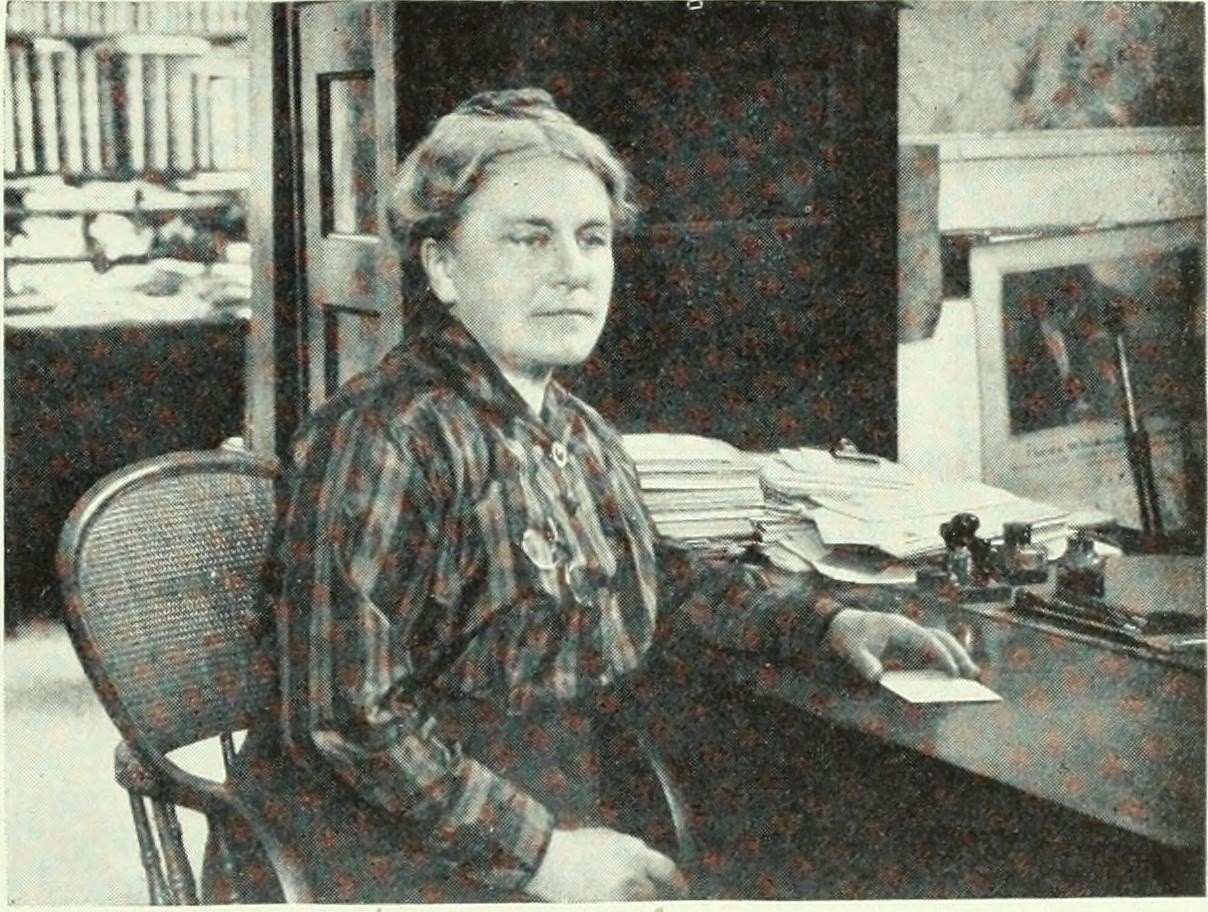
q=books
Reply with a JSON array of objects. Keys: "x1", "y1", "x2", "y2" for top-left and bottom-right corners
[
  {"x1": 751, "y1": 448, "x2": 1069, "y2": 587},
  {"x1": 617, "y1": 432, "x2": 761, "y2": 560},
  {"x1": 0, "y1": 0, "x2": 253, "y2": 171}
]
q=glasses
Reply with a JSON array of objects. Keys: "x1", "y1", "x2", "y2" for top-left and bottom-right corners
[{"x1": 516, "y1": 519, "x2": 607, "y2": 617}]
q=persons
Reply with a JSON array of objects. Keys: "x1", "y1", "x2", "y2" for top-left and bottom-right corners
[{"x1": 197, "y1": 88, "x2": 983, "y2": 906}]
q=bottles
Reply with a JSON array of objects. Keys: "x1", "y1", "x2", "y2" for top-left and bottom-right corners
[
  {"x1": 1054, "y1": 529, "x2": 1104, "y2": 598},
  {"x1": 992, "y1": 523, "x2": 1048, "y2": 586}
]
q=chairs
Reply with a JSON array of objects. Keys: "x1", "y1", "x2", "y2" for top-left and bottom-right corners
[{"x1": 56, "y1": 449, "x2": 698, "y2": 904}]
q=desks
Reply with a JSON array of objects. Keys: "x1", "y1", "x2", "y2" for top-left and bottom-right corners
[{"x1": 675, "y1": 544, "x2": 1204, "y2": 895}]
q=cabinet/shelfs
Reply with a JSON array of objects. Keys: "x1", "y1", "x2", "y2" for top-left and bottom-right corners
[{"x1": 4, "y1": 309, "x2": 254, "y2": 626}]
